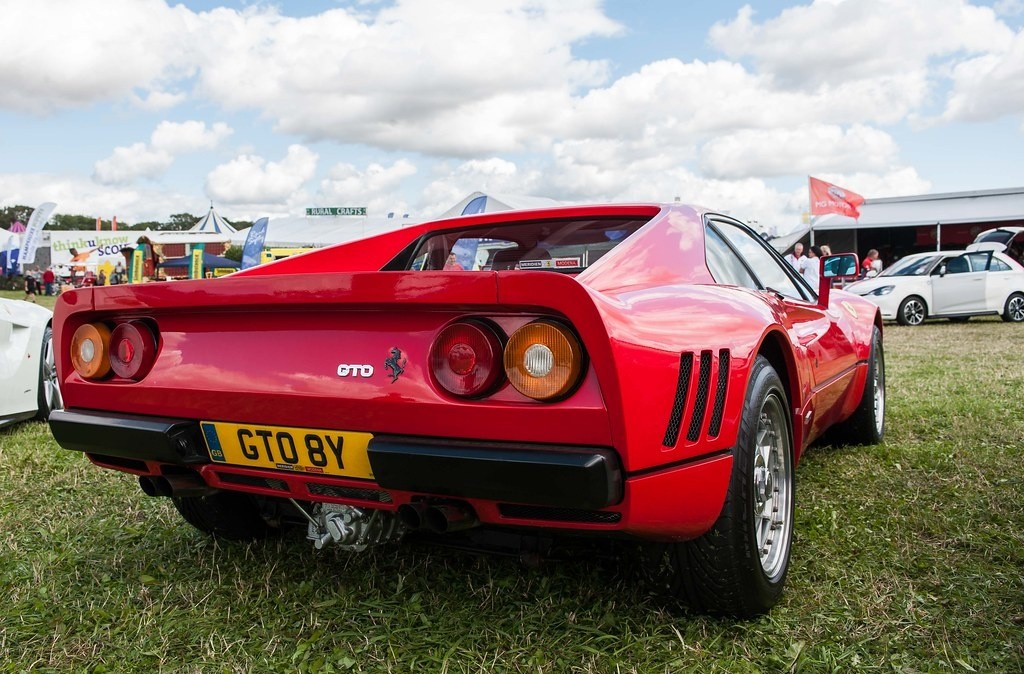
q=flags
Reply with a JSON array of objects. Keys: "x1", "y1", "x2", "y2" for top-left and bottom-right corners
[{"x1": 810, "y1": 176, "x2": 865, "y2": 220}]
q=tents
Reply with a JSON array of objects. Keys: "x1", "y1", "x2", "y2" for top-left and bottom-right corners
[
  {"x1": 187, "y1": 199, "x2": 238, "y2": 234},
  {"x1": 7, "y1": 220, "x2": 26, "y2": 233}
]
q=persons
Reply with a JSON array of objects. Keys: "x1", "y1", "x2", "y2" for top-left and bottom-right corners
[
  {"x1": 69, "y1": 245, "x2": 103, "y2": 262},
  {"x1": 783, "y1": 243, "x2": 832, "y2": 296},
  {"x1": 98, "y1": 261, "x2": 125, "y2": 286},
  {"x1": 862, "y1": 249, "x2": 879, "y2": 278},
  {"x1": 442, "y1": 252, "x2": 464, "y2": 271},
  {"x1": 21, "y1": 263, "x2": 93, "y2": 303}
]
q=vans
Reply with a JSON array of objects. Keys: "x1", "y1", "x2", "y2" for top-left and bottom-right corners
[{"x1": 972, "y1": 226, "x2": 1024, "y2": 267}]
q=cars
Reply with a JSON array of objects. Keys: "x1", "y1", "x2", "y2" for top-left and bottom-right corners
[
  {"x1": 841, "y1": 241, "x2": 1024, "y2": 326},
  {"x1": 0, "y1": 295, "x2": 64, "y2": 430}
]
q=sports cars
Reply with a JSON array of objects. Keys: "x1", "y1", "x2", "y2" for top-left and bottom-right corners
[{"x1": 47, "y1": 199, "x2": 888, "y2": 625}]
